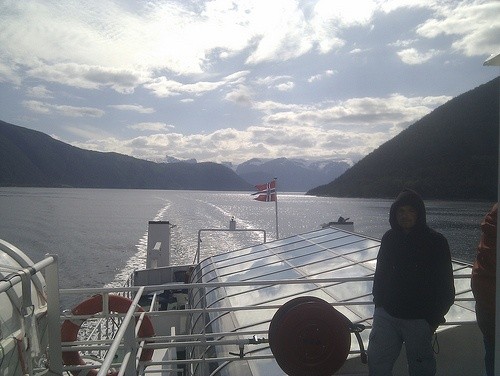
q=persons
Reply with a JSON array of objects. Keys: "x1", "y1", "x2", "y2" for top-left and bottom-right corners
[
  {"x1": 367, "y1": 186, "x2": 455, "y2": 376},
  {"x1": 471, "y1": 202, "x2": 497, "y2": 376}
]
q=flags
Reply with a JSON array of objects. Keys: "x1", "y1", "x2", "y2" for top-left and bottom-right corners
[{"x1": 254, "y1": 181, "x2": 276, "y2": 202}]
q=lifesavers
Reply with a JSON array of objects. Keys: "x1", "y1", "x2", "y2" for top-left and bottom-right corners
[{"x1": 62, "y1": 295, "x2": 154, "y2": 376}]
